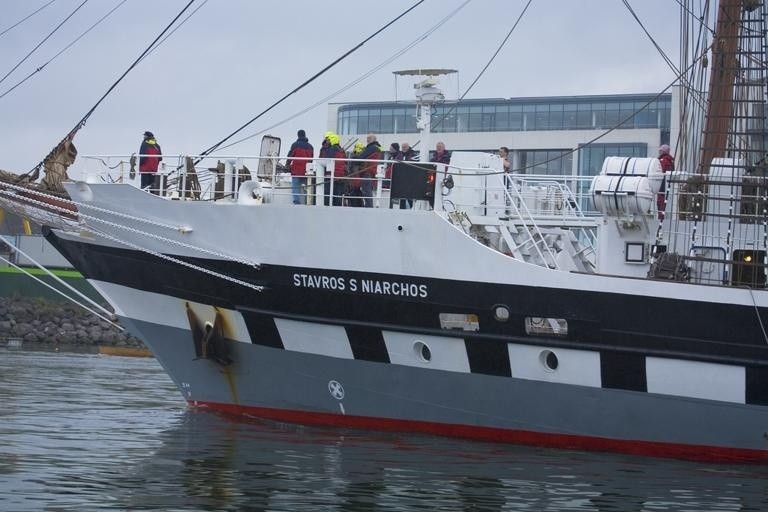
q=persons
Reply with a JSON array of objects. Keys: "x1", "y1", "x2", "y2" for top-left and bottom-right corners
[
  {"x1": 499, "y1": 146, "x2": 511, "y2": 190},
  {"x1": 319, "y1": 130, "x2": 450, "y2": 210},
  {"x1": 285, "y1": 130, "x2": 314, "y2": 205},
  {"x1": 658, "y1": 144, "x2": 675, "y2": 219},
  {"x1": 139, "y1": 131, "x2": 163, "y2": 193}
]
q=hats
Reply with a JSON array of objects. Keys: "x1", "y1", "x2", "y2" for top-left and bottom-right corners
[{"x1": 143, "y1": 131, "x2": 153, "y2": 138}]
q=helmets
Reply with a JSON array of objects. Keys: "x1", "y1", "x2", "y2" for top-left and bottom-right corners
[{"x1": 325, "y1": 131, "x2": 339, "y2": 145}]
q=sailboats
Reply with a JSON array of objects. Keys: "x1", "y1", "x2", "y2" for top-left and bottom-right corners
[{"x1": 0, "y1": 0, "x2": 767, "y2": 467}]
